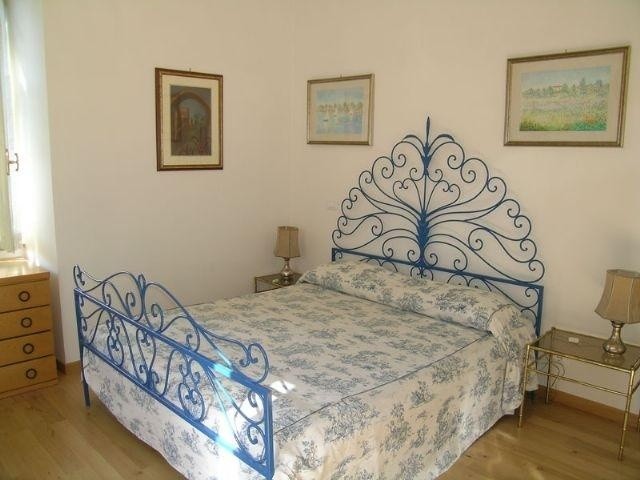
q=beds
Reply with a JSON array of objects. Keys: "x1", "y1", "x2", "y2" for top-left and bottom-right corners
[{"x1": 73, "y1": 117, "x2": 545, "y2": 480}]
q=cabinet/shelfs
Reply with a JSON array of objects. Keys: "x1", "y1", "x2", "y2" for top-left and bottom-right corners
[{"x1": 0, "y1": 257, "x2": 59, "y2": 399}]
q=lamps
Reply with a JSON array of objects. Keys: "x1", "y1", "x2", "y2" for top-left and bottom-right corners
[
  {"x1": 272, "y1": 226, "x2": 301, "y2": 278},
  {"x1": 593, "y1": 269, "x2": 640, "y2": 354}
]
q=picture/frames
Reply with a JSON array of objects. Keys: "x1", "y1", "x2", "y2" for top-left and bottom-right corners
[
  {"x1": 503, "y1": 44, "x2": 631, "y2": 147},
  {"x1": 155, "y1": 67, "x2": 223, "y2": 171},
  {"x1": 306, "y1": 73, "x2": 376, "y2": 146}
]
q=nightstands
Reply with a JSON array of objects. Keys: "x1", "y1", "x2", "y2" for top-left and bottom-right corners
[{"x1": 255, "y1": 271, "x2": 303, "y2": 293}]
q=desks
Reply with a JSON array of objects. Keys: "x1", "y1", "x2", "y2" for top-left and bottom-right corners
[{"x1": 517, "y1": 327, "x2": 640, "y2": 460}]
